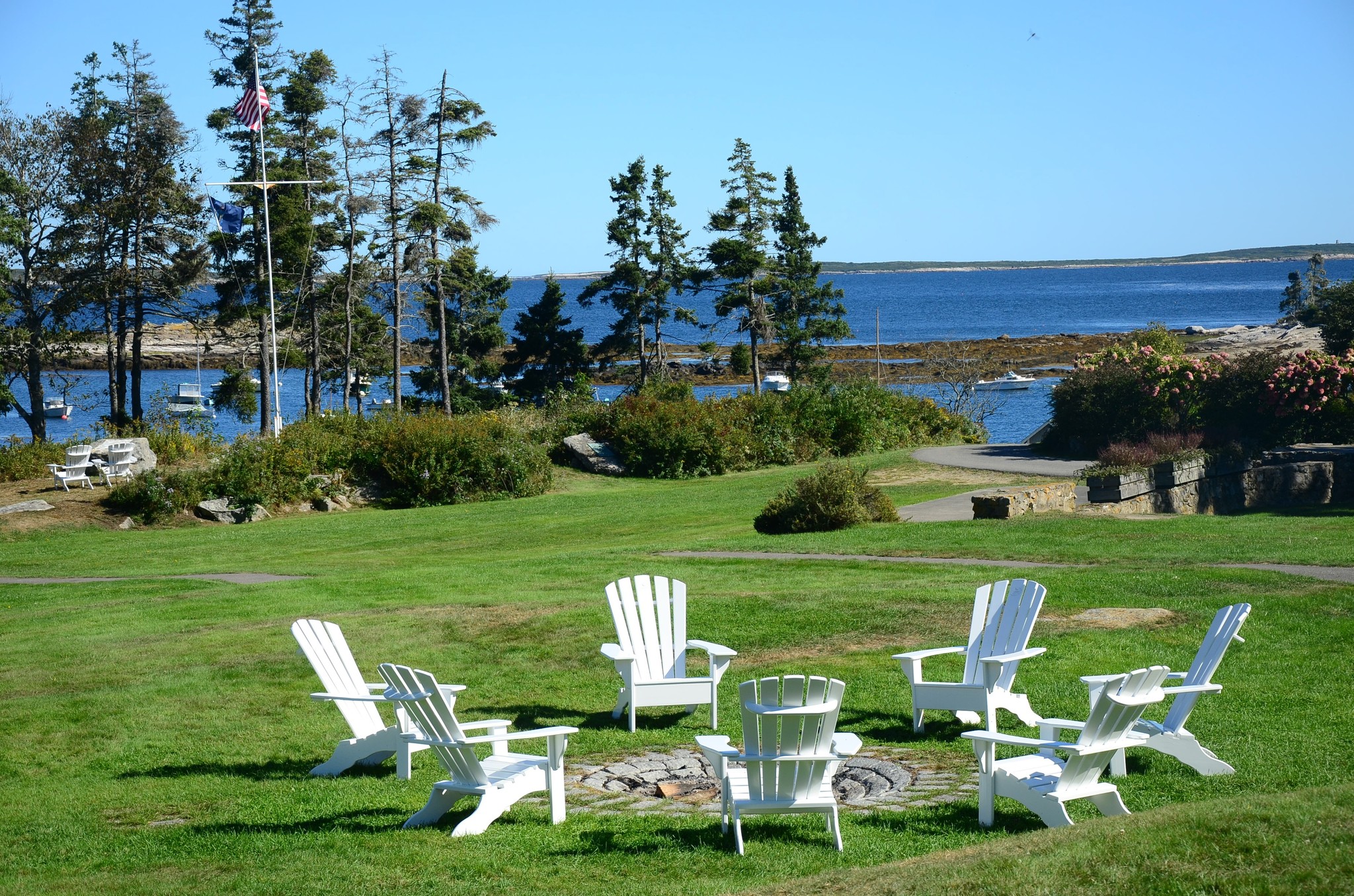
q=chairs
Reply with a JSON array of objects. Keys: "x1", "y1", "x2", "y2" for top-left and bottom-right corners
[
  {"x1": 600, "y1": 575, "x2": 737, "y2": 734},
  {"x1": 377, "y1": 663, "x2": 579, "y2": 838},
  {"x1": 1079, "y1": 603, "x2": 1251, "y2": 778},
  {"x1": 695, "y1": 675, "x2": 862, "y2": 856},
  {"x1": 291, "y1": 619, "x2": 466, "y2": 778},
  {"x1": 46, "y1": 445, "x2": 94, "y2": 493},
  {"x1": 92, "y1": 443, "x2": 137, "y2": 487},
  {"x1": 891, "y1": 579, "x2": 1047, "y2": 735},
  {"x1": 961, "y1": 665, "x2": 1171, "y2": 827}
]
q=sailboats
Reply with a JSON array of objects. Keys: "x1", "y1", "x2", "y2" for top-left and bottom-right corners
[{"x1": 167, "y1": 334, "x2": 215, "y2": 418}]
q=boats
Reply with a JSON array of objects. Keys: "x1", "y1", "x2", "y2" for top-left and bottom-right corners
[
  {"x1": 211, "y1": 372, "x2": 262, "y2": 393},
  {"x1": 761, "y1": 368, "x2": 790, "y2": 391},
  {"x1": 365, "y1": 397, "x2": 395, "y2": 409},
  {"x1": 348, "y1": 367, "x2": 371, "y2": 395},
  {"x1": 18, "y1": 395, "x2": 73, "y2": 417},
  {"x1": 969, "y1": 361, "x2": 1036, "y2": 390},
  {"x1": 472, "y1": 380, "x2": 508, "y2": 401}
]
